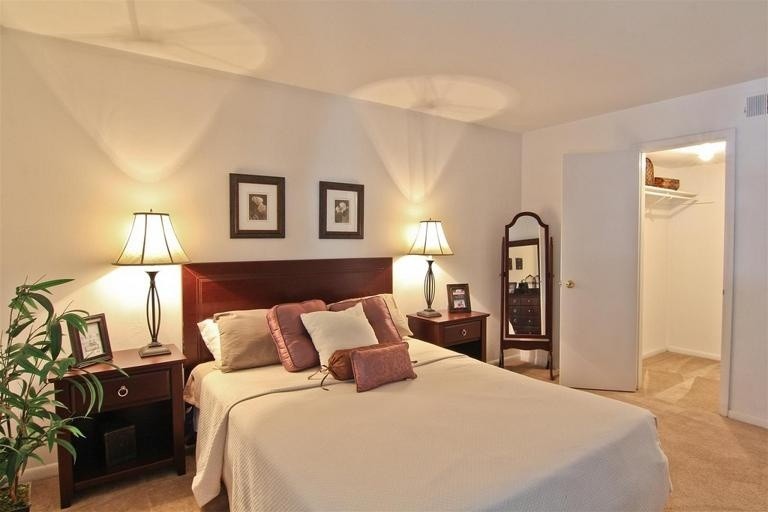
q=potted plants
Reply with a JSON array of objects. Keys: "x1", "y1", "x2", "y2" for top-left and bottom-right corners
[{"x1": 0, "y1": 278, "x2": 129, "y2": 512}]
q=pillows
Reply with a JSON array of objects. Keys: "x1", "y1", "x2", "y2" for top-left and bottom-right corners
[
  {"x1": 215, "y1": 310, "x2": 280, "y2": 373},
  {"x1": 375, "y1": 294, "x2": 414, "y2": 339},
  {"x1": 298, "y1": 302, "x2": 380, "y2": 372},
  {"x1": 350, "y1": 345, "x2": 413, "y2": 392},
  {"x1": 329, "y1": 344, "x2": 396, "y2": 382},
  {"x1": 266, "y1": 299, "x2": 329, "y2": 373},
  {"x1": 333, "y1": 294, "x2": 404, "y2": 346},
  {"x1": 198, "y1": 320, "x2": 220, "y2": 362}
]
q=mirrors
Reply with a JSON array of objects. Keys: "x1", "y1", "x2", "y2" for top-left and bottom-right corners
[{"x1": 497, "y1": 211, "x2": 560, "y2": 383}]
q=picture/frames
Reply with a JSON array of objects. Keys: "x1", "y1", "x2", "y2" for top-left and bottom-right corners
[
  {"x1": 319, "y1": 182, "x2": 365, "y2": 240},
  {"x1": 66, "y1": 314, "x2": 115, "y2": 368},
  {"x1": 229, "y1": 173, "x2": 286, "y2": 240},
  {"x1": 446, "y1": 283, "x2": 472, "y2": 315}
]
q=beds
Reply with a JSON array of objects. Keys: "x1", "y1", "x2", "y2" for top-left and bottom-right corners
[{"x1": 182, "y1": 257, "x2": 655, "y2": 512}]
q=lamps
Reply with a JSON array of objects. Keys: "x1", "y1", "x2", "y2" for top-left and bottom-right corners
[
  {"x1": 407, "y1": 220, "x2": 456, "y2": 318},
  {"x1": 114, "y1": 212, "x2": 189, "y2": 358}
]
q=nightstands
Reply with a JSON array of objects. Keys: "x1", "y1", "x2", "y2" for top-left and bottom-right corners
[
  {"x1": 407, "y1": 311, "x2": 490, "y2": 362},
  {"x1": 50, "y1": 344, "x2": 187, "y2": 508}
]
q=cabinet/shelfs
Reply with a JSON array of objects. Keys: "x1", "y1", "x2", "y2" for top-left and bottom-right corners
[{"x1": 508, "y1": 289, "x2": 541, "y2": 339}]
